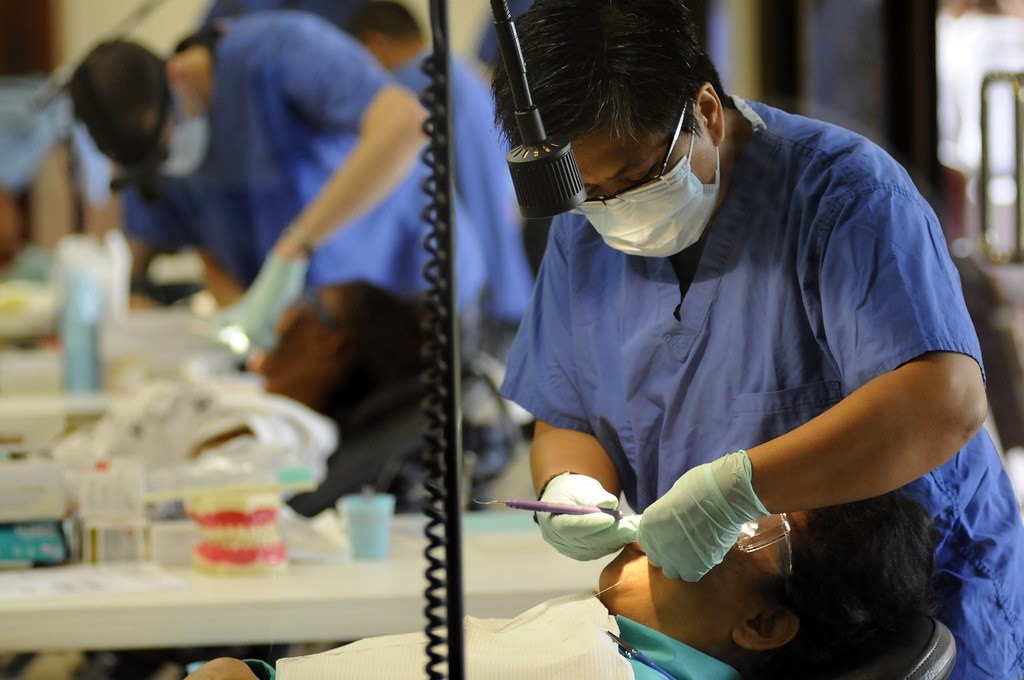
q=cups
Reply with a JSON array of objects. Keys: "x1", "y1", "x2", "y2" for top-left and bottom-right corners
[{"x1": 337, "y1": 491, "x2": 393, "y2": 560}]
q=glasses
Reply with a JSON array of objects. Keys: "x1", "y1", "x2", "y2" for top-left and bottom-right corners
[
  {"x1": 579, "y1": 96, "x2": 695, "y2": 212},
  {"x1": 737, "y1": 513, "x2": 794, "y2": 603},
  {"x1": 118, "y1": 78, "x2": 174, "y2": 178}
]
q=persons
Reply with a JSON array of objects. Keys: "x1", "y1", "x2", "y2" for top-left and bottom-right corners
[
  {"x1": 184, "y1": 492, "x2": 931, "y2": 680},
  {"x1": 488, "y1": 0, "x2": 1024, "y2": 680},
  {"x1": 0, "y1": 0, "x2": 536, "y2": 525}
]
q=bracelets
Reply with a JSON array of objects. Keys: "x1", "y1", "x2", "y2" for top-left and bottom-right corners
[
  {"x1": 533, "y1": 471, "x2": 580, "y2": 525},
  {"x1": 283, "y1": 224, "x2": 314, "y2": 259}
]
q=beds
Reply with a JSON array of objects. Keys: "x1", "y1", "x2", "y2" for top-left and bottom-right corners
[
  {"x1": 838, "y1": 610, "x2": 957, "y2": 680},
  {"x1": 63, "y1": 367, "x2": 514, "y2": 680}
]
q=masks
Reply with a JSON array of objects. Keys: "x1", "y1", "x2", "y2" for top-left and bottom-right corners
[
  {"x1": 577, "y1": 121, "x2": 721, "y2": 258},
  {"x1": 163, "y1": 121, "x2": 210, "y2": 179}
]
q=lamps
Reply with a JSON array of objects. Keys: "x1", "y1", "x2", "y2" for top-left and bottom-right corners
[{"x1": 416, "y1": 0, "x2": 587, "y2": 680}]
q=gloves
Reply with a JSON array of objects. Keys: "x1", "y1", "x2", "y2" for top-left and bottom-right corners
[
  {"x1": 636, "y1": 453, "x2": 770, "y2": 587},
  {"x1": 221, "y1": 256, "x2": 304, "y2": 348},
  {"x1": 533, "y1": 472, "x2": 642, "y2": 563}
]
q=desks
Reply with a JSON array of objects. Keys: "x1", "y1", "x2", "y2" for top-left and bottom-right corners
[{"x1": 0, "y1": 515, "x2": 620, "y2": 680}]
